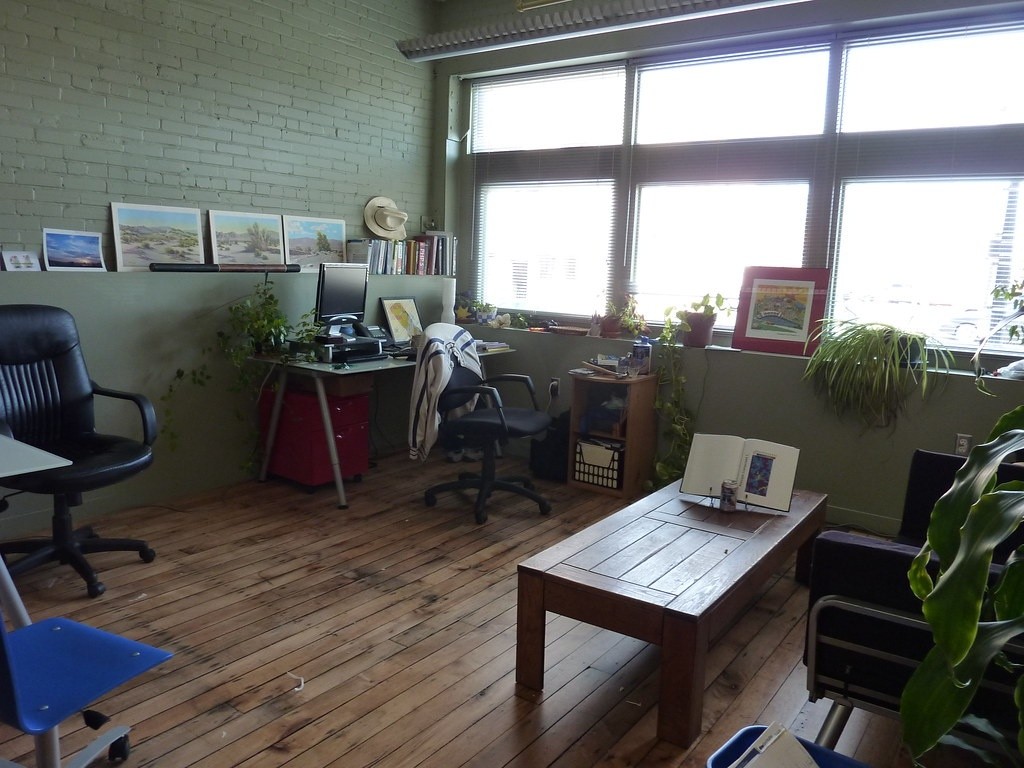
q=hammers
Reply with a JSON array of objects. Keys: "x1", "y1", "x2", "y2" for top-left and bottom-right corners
[{"x1": 581, "y1": 361, "x2": 629, "y2": 380}]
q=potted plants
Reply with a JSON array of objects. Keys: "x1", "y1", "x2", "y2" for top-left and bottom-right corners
[
  {"x1": 472, "y1": 298, "x2": 497, "y2": 325},
  {"x1": 800, "y1": 314, "x2": 957, "y2": 442},
  {"x1": 156, "y1": 280, "x2": 330, "y2": 479},
  {"x1": 600, "y1": 289, "x2": 652, "y2": 340},
  {"x1": 641, "y1": 291, "x2": 737, "y2": 493}
]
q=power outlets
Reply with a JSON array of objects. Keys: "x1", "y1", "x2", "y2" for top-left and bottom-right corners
[{"x1": 549, "y1": 377, "x2": 560, "y2": 397}]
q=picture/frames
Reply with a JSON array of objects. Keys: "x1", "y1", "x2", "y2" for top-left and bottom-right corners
[
  {"x1": 283, "y1": 215, "x2": 347, "y2": 271},
  {"x1": 110, "y1": 202, "x2": 206, "y2": 272},
  {"x1": 208, "y1": 210, "x2": 285, "y2": 266},
  {"x1": 731, "y1": 266, "x2": 831, "y2": 358}
]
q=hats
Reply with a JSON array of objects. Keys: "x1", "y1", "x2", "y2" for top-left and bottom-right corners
[{"x1": 364, "y1": 197, "x2": 408, "y2": 241}]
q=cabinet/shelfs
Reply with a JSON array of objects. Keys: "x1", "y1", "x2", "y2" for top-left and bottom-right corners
[{"x1": 567, "y1": 368, "x2": 657, "y2": 500}]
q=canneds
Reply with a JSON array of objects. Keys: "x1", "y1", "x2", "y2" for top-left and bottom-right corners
[{"x1": 720, "y1": 479, "x2": 738, "y2": 512}]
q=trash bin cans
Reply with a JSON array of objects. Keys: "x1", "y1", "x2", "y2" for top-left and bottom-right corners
[{"x1": 706, "y1": 725, "x2": 870, "y2": 768}]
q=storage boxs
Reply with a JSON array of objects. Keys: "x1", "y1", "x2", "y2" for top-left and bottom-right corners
[{"x1": 258, "y1": 386, "x2": 371, "y2": 493}]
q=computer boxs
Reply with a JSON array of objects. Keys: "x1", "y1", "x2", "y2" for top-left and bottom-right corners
[{"x1": 288, "y1": 339, "x2": 382, "y2": 363}]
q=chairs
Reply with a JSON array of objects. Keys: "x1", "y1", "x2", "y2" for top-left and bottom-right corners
[
  {"x1": 412, "y1": 322, "x2": 552, "y2": 523},
  {"x1": 804, "y1": 447, "x2": 1024, "y2": 752},
  {"x1": 0, "y1": 303, "x2": 157, "y2": 597},
  {"x1": 0, "y1": 611, "x2": 175, "y2": 768}
]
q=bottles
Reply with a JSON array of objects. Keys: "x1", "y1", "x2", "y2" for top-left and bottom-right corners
[{"x1": 635, "y1": 335, "x2": 653, "y2": 375}]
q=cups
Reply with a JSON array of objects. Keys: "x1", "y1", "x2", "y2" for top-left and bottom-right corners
[{"x1": 615, "y1": 363, "x2": 640, "y2": 379}]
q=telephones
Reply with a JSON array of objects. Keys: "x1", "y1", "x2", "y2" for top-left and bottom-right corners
[{"x1": 352, "y1": 321, "x2": 393, "y2": 347}]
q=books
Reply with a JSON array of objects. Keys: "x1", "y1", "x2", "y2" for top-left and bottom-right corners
[
  {"x1": 477, "y1": 341, "x2": 509, "y2": 353},
  {"x1": 680, "y1": 432, "x2": 801, "y2": 513},
  {"x1": 346, "y1": 230, "x2": 458, "y2": 276}
]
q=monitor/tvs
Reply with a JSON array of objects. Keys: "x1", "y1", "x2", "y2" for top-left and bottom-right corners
[{"x1": 314, "y1": 262, "x2": 370, "y2": 341}]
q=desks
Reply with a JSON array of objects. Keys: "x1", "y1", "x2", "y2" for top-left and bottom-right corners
[
  {"x1": 0, "y1": 433, "x2": 73, "y2": 628},
  {"x1": 515, "y1": 472, "x2": 829, "y2": 750},
  {"x1": 246, "y1": 342, "x2": 517, "y2": 510}
]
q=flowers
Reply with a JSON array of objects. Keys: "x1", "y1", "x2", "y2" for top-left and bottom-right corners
[{"x1": 455, "y1": 295, "x2": 472, "y2": 321}]
